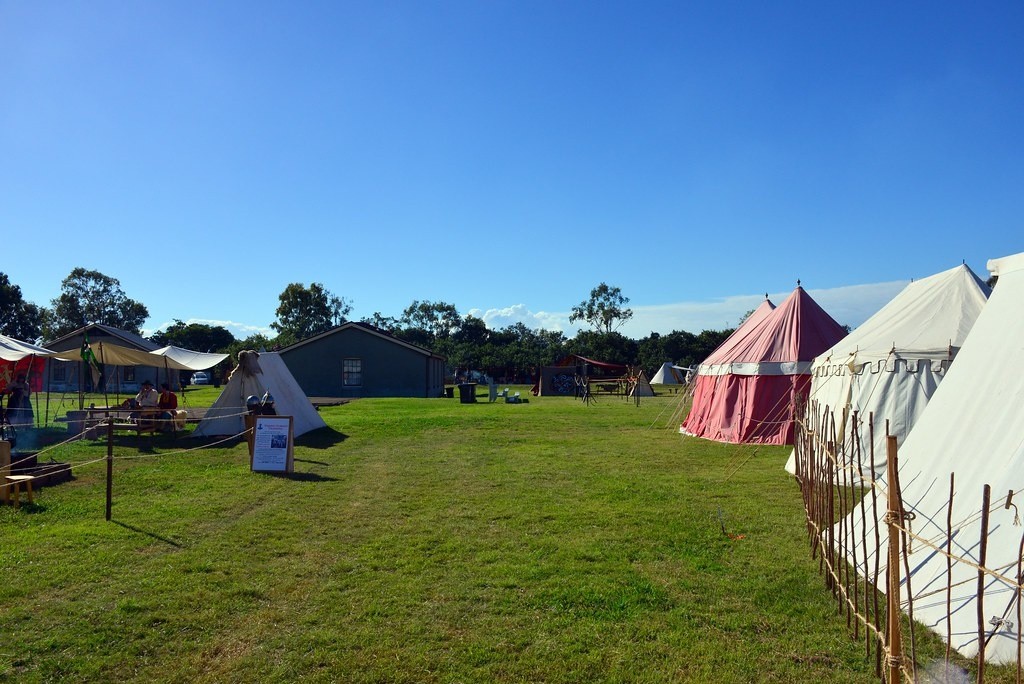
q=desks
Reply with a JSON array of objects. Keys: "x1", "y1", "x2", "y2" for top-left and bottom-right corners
[{"x1": 80, "y1": 406, "x2": 177, "y2": 441}]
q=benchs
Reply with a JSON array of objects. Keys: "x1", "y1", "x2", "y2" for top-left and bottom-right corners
[{"x1": 80, "y1": 417, "x2": 177, "y2": 431}]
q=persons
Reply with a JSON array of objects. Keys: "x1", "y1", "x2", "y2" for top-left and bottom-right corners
[
  {"x1": 158, "y1": 383, "x2": 178, "y2": 432},
  {"x1": 2, "y1": 372, "x2": 34, "y2": 432},
  {"x1": 129, "y1": 380, "x2": 159, "y2": 427}
]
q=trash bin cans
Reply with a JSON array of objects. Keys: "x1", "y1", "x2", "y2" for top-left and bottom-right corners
[
  {"x1": 458, "y1": 383, "x2": 477, "y2": 403},
  {"x1": 444, "y1": 387, "x2": 454, "y2": 398}
]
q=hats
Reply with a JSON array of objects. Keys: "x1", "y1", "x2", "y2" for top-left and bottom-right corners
[{"x1": 141, "y1": 379, "x2": 154, "y2": 386}]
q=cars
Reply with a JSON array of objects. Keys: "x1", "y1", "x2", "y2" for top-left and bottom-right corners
[
  {"x1": 190, "y1": 372, "x2": 208, "y2": 384},
  {"x1": 685, "y1": 364, "x2": 698, "y2": 385}
]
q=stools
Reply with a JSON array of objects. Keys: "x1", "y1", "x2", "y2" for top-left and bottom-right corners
[{"x1": 4, "y1": 474, "x2": 35, "y2": 508}]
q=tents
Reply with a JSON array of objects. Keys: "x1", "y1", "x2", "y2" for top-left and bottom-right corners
[
  {"x1": 626, "y1": 252, "x2": 1024, "y2": 672},
  {"x1": 189, "y1": 350, "x2": 326, "y2": 441}
]
